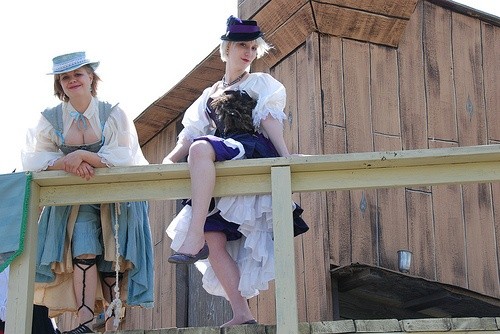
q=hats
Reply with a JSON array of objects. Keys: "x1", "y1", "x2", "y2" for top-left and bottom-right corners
[
  {"x1": 45, "y1": 52, "x2": 99, "y2": 75},
  {"x1": 221, "y1": 15, "x2": 266, "y2": 41}
]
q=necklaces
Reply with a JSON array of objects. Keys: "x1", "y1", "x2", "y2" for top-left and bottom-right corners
[{"x1": 222, "y1": 70, "x2": 247, "y2": 86}]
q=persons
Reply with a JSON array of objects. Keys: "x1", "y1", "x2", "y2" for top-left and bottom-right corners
[
  {"x1": 160, "y1": 15, "x2": 310, "y2": 328},
  {"x1": 19, "y1": 51, "x2": 156, "y2": 333}
]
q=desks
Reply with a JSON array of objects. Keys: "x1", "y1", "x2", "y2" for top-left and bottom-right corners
[{"x1": 330, "y1": 262, "x2": 500, "y2": 321}]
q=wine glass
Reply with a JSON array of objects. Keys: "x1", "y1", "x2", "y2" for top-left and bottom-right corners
[{"x1": 398, "y1": 250, "x2": 413, "y2": 274}]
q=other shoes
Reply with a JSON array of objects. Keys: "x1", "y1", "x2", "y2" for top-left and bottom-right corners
[
  {"x1": 62, "y1": 259, "x2": 98, "y2": 334},
  {"x1": 168, "y1": 240, "x2": 209, "y2": 264},
  {"x1": 240, "y1": 320, "x2": 258, "y2": 324},
  {"x1": 100, "y1": 271, "x2": 124, "y2": 334}
]
